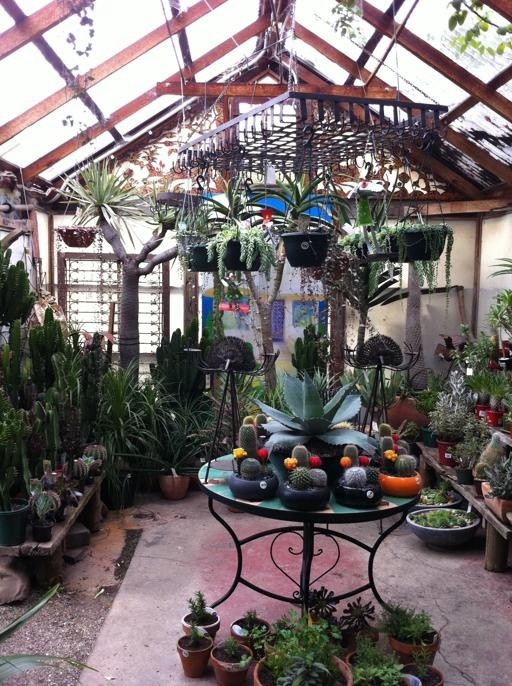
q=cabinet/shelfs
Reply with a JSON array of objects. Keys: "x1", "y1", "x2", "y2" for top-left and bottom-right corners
[
  {"x1": 1, "y1": 469, "x2": 107, "y2": 577},
  {"x1": 415, "y1": 427, "x2": 512, "y2": 574}
]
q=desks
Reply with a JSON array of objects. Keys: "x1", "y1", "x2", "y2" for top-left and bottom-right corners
[{"x1": 194, "y1": 449, "x2": 422, "y2": 610}]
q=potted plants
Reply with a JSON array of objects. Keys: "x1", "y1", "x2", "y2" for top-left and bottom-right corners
[
  {"x1": 0, "y1": 251, "x2": 153, "y2": 546},
  {"x1": 174, "y1": 586, "x2": 447, "y2": 686},
  {"x1": 409, "y1": 289, "x2": 512, "y2": 546},
  {"x1": 148, "y1": 139, "x2": 453, "y2": 512}
]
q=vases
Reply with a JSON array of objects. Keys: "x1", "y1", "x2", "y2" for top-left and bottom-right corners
[{"x1": 54, "y1": 226, "x2": 99, "y2": 247}]
